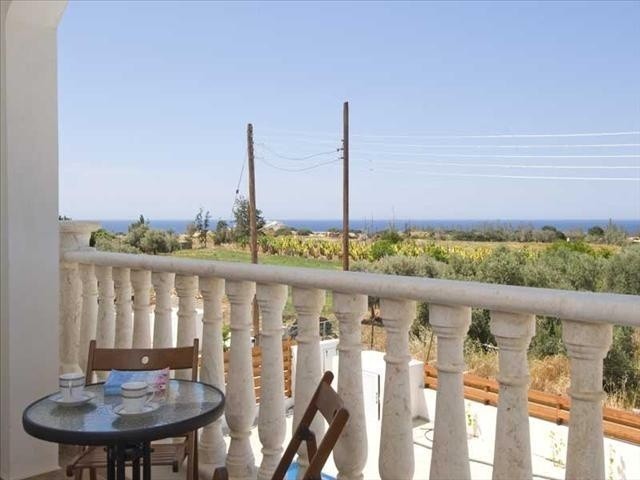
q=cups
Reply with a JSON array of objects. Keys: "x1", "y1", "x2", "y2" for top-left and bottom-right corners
[
  {"x1": 59, "y1": 373, "x2": 86, "y2": 400},
  {"x1": 121, "y1": 382, "x2": 155, "y2": 412}
]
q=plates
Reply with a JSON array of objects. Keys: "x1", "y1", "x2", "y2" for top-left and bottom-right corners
[
  {"x1": 114, "y1": 402, "x2": 160, "y2": 417},
  {"x1": 51, "y1": 391, "x2": 95, "y2": 407}
]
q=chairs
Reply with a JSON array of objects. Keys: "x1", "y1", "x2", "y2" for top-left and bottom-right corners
[{"x1": 64, "y1": 340, "x2": 350, "y2": 480}]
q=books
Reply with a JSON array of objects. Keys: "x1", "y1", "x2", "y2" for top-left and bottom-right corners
[{"x1": 105, "y1": 367, "x2": 169, "y2": 396}]
